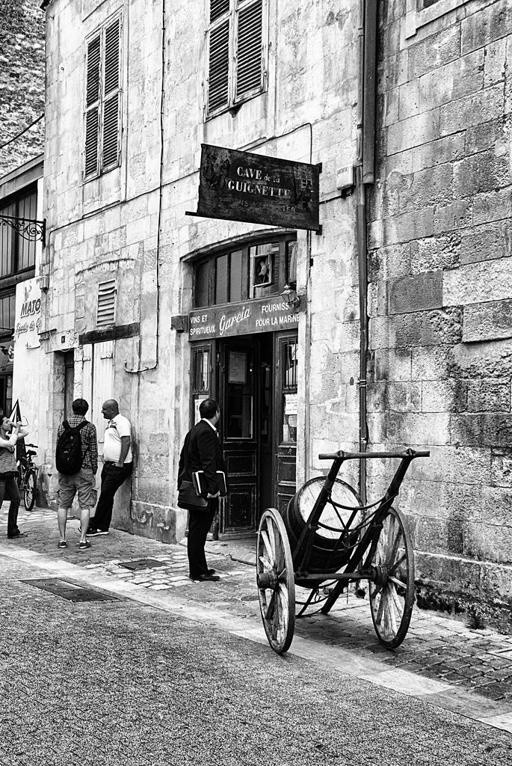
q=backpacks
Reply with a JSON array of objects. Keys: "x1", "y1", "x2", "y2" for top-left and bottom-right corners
[{"x1": 55, "y1": 419, "x2": 90, "y2": 476}]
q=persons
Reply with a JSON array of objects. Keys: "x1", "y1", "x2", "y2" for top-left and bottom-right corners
[
  {"x1": 55, "y1": 398, "x2": 100, "y2": 548},
  {"x1": 176, "y1": 397, "x2": 226, "y2": 581},
  {"x1": 76, "y1": 397, "x2": 135, "y2": 536},
  {"x1": 0, "y1": 414, "x2": 32, "y2": 539}
]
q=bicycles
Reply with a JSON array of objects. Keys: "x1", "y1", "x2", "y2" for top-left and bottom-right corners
[{"x1": 9, "y1": 443, "x2": 40, "y2": 515}]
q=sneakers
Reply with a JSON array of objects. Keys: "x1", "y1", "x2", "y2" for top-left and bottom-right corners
[
  {"x1": 8, "y1": 533, "x2": 26, "y2": 538},
  {"x1": 57, "y1": 541, "x2": 67, "y2": 548},
  {"x1": 79, "y1": 539, "x2": 91, "y2": 549},
  {"x1": 78, "y1": 522, "x2": 110, "y2": 537}
]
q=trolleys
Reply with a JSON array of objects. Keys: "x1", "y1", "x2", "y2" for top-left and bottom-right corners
[{"x1": 245, "y1": 444, "x2": 443, "y2": 667}]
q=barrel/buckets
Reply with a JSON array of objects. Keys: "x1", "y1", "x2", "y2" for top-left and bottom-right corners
[{"x1": 281, "y1": 476, "x2": 364, "y2": 588}]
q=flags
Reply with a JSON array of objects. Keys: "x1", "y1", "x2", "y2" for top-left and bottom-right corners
[{"x1": 9, "y1": 400, "x2": 28, "y2": 465}]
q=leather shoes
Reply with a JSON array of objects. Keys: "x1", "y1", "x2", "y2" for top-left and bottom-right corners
[{"x1": 189, "y1": 569, "x2": 220, "y2": 582}]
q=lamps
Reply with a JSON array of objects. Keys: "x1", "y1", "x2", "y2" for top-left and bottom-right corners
[{"x1": 278, "y1": 282, "x2": 306, "y2": 309}]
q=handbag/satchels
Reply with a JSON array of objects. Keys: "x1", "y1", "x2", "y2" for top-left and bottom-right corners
[{"x1": 177, "y1": 479, "x2": 210, "y2": 511}]
